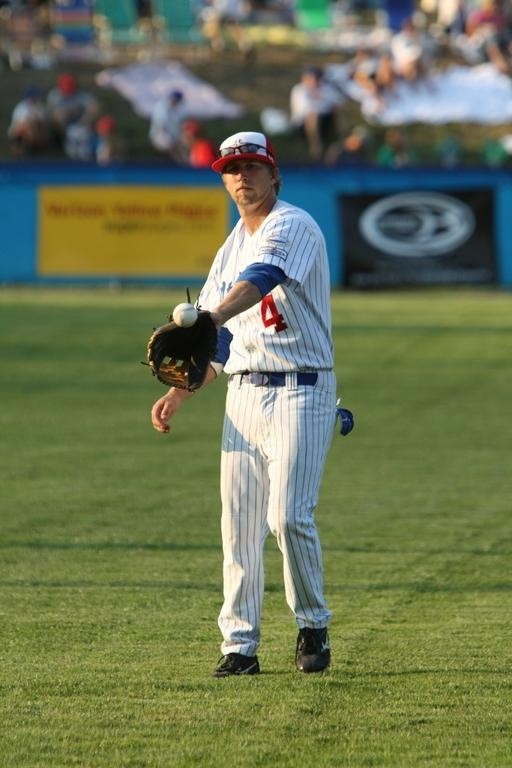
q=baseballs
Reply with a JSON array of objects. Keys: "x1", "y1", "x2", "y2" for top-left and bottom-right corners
[{"x1": 173, "y1": 302, "x2": 197, "y2": 327}]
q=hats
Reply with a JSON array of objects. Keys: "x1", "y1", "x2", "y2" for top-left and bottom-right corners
[{"x1": 212, "y1": 131, "x2": 277, "y2": 167}]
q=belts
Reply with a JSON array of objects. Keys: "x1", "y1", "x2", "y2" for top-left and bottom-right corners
[{"x1": 230, "y1": 372, "x2": 317, "y2": 386}]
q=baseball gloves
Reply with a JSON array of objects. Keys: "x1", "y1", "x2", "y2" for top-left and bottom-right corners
[{"x1": 140, "y1": 289, "x2": 219, "y2": 393}]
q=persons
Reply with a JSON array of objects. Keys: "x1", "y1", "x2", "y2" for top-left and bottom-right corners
[
  {"x1": 0, "y1": 0, "x2": 512, "y2": 167},
  {"x1": 151, "y1": 129, "x2": 340, "y2": 674}
]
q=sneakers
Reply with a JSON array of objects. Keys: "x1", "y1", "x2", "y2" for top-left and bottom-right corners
[
  {"x1": 297, "y1": 627, "x2": 330, "y2": 673},
  {"x1": 211, "y1": 652, "x2": 259, "y2": 677}
]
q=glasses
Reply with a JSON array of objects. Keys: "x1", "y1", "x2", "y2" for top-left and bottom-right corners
[{"x1": 216, "y1": 143, "x2": 275, "y2": 160}]
